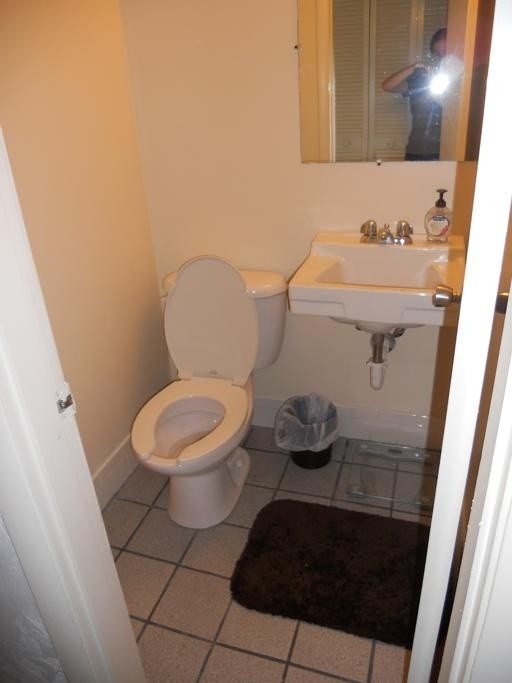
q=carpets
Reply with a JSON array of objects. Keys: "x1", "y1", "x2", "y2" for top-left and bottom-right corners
[{"x1": 228, "y1": 496, "x2": 432, "y2": 653}]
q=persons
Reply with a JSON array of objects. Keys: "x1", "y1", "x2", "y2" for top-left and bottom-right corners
[{"x1": 380, "y1": 28, "x2": 445, "y2": 159}]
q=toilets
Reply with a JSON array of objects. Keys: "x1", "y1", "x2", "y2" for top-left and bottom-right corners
[{"x1": 129, "y1": 255, "x2": 285, "y2": 530}]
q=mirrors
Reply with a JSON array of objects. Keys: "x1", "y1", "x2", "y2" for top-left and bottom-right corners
[{"x1": 295, "y1": 0, "x2": 496, "y2": 166}]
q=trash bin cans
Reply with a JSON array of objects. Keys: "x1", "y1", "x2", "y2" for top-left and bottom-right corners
[{"x1": 282, "y1": 396, "x2": 337, "y2": 469}]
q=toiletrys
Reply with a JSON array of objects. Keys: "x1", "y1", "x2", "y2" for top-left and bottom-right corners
[{"x1": 424, "y1": 189, "x2": 454, "y2": 242}]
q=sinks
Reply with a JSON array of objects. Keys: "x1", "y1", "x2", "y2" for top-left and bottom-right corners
[{"x1": 288, "y1": 232, "x2": 465, "y2": 327}]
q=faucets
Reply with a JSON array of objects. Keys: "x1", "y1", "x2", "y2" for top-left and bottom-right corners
[{"x1": 377, "y1": 227, "x2": 394, "y2": 244}]
